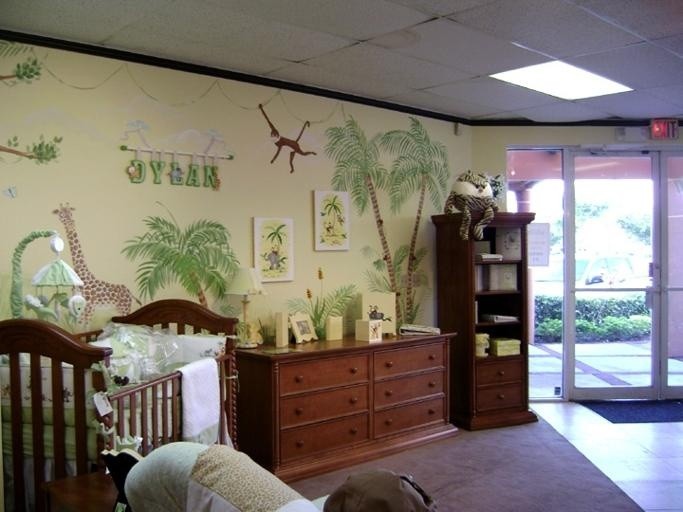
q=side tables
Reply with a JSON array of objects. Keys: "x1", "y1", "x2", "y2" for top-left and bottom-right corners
[{"x1": 32, "y1": 467, "x2": 133, "y2": 512}]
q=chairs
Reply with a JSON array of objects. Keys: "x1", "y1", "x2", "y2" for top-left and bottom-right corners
[{"x1": 114, "y1": 440, "x2": 442, "y2": 512}]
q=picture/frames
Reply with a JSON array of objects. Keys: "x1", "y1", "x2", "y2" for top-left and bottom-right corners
[{"x1": 101, "y1": 449, "x2": 144, "y2": 512}]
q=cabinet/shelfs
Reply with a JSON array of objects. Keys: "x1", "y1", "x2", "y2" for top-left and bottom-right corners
[
  {"x1": 235, "y1": 331, "x2": 457, "y2": 485},
  {"x1": 430, "y1": 210, "x2": 536, "y2": 432}
]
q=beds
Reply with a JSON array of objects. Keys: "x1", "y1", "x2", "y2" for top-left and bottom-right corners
[{"x1": 0, "y1": 300, "x2": 241, "y2": 512}]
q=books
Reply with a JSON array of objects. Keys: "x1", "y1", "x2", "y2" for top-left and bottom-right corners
[{"x1": 472, "y1": 226, "x2": 522, "y2": 325}]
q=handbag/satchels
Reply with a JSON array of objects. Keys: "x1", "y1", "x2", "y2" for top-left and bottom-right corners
[{"x1": 324, "y1": 469, "x2": 437, "y2": 512}]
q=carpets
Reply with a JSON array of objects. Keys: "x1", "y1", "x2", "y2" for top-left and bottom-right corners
[{"x1": 575, "y1": 399, "x2": 683, "y2": 424}]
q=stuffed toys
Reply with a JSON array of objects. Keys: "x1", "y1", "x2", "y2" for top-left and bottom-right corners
[{"x1": 444, "y1": 169, "x2": 505, "y2": 242}]
